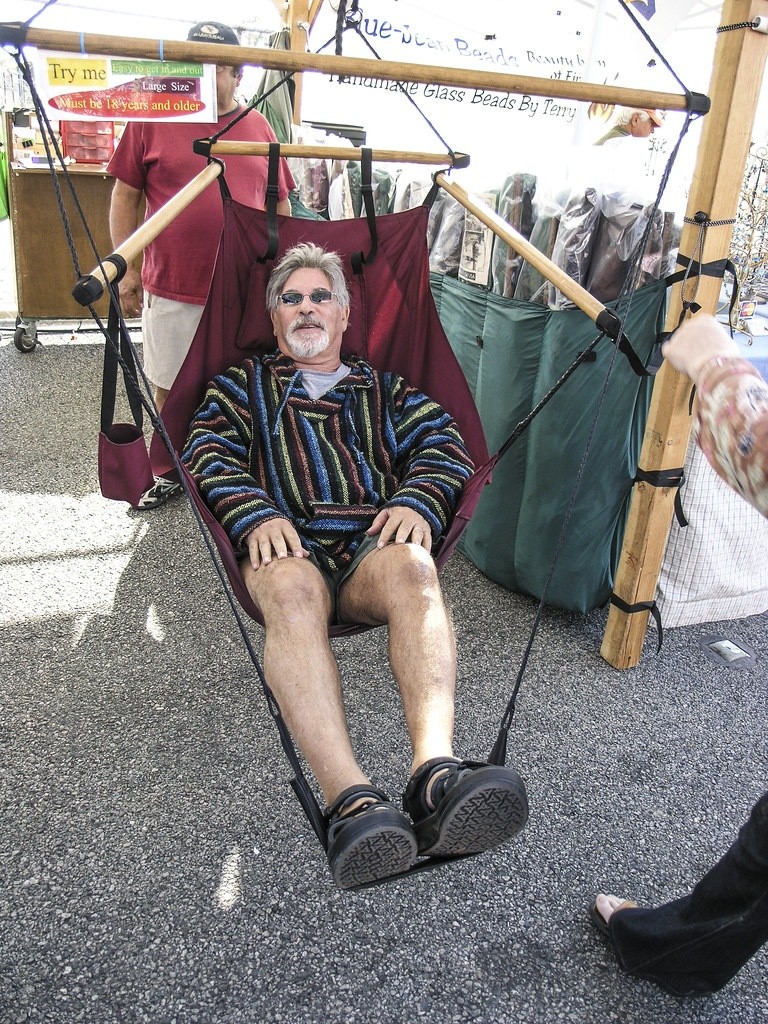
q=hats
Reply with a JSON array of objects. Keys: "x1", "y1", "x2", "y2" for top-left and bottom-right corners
[
  {"x1": 637, "y1": 106, "x2": 662, "y2": 127},
  {"x1": 186, "y1": 21, "x2": 239, "y2": 45}
]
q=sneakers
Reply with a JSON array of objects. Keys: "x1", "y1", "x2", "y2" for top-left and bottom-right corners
[{"x1": 137, "y1": 476, "x2": 186, "y2": 509}]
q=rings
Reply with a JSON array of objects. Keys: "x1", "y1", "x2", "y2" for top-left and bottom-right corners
[{"x1": 413, "y1": 527, "x2": 423, "y2": 530}]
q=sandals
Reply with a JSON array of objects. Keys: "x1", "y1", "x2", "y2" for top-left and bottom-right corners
[
  {"x1": 589, "y1": 896, "x2": 640, "y2": 936},
  {"x1": 402, "y1": 756, "x2": 529, "y2": 856},
  {"x1": 324, "y1": 783, "x2": 418, "y2": 890}
]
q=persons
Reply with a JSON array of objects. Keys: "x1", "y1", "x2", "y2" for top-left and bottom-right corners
[
  {"x1": 591, "y1": 314, "x2": 768, "y2": 995},
  {"x1": 106, "y1": 21, "x2": 296, "y2": 508},
  {"x1": 595, "y1": 108, "x2": 661, "y2": 147},
  {"x1": 180, "y1": 241, "x2": 528, "y2": 889}
]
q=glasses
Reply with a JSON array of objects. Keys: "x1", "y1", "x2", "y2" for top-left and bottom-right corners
[
  {"x1": 216, "y1": 65, "x2": 235, "y2": 73},
  {"x1": 277, "y1": 291, "x2": 337, "y2": 305}
]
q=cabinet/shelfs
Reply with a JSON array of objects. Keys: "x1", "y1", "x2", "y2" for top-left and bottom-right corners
[{"x1": 5, "y1": 109, "x2": 148, "y2": 351}]
